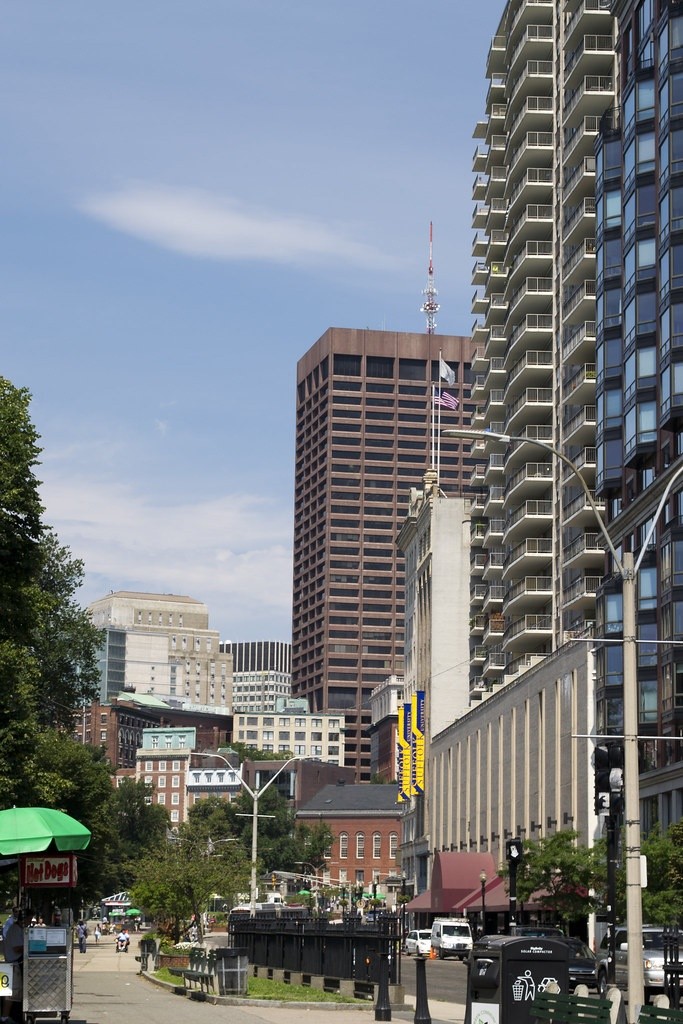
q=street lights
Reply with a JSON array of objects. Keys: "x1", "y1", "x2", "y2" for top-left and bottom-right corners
[
  {"x1": 294, "y1": 861, "x2": 330, "y2": 908},
  {"x1": 373, "y1": 875, "x2": 379, "y2": 924},
  {"x1": 441, "y1": 427, "x2": 683, "y2": 1023},
  {"x1": 341, "y1": 881, "x2": 346, "y2": 916},
  {"x1": 189, "y1": 752, "x2": 326, "y2": 920},
  {"x1": 358, "y1": 881, "x2": 363, "y2": 905},
  {"x1": 401, "y1": 869, "x2": 408, "y2": 953},
  {"x1": 478, "y1": 869, "x2": 487, "y2": 936},
  {"x1": 352, "y1": 883, "x2": 357, "y2": 899}
]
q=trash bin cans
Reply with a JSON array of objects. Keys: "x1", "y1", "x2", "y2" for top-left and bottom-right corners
[
  {"x1": 140, "y1": 940, "x2": 156, "y2": 970},
  {"x1": 463, "y1": 933, "x2": 573, "y2": 1024},
  {"x1": 216, "y1": 947, "x2": 250, "y2": 996}
]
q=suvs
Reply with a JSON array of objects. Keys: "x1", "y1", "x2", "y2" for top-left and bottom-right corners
[
  {"x1": 472, "y1": 924, "x2": 608, "y2": 995},
  {"x1": 595, "y1": 923, "x2": 683, "y2": 1005}
]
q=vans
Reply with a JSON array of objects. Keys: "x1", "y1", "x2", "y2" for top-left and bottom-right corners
[{"x1": 432, "y1": 917, "x2": 474, "y2": 961}]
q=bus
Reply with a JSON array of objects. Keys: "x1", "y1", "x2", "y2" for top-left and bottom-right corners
[{"x1": 230, "y1": 901, "x2": 309, "y2": 914}]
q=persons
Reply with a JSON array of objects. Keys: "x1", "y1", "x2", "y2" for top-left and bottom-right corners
[
  {"x1": 77, "y1": 920, "x2": 87, "y2": 953},
  {"x1": 94, "y1": 923, "x2": 101, "y2": 944},
  {"x1": 114, "y1": 929, "x2": 130, "y2": 950},
  {"x1": 2, "y1": 907, "x2": 47, "y2": 979},
  {"x1": 134, "y1": 915, "x2": 141, "y2": 932}
]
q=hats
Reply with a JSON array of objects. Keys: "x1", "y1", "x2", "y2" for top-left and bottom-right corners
[{"x1": 79, "y1": 919, "x2": 83, "y2": 922}]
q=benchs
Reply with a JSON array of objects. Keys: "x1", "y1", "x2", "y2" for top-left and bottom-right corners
[
  {"x1": 638, "y1": 1006, "x2": 683, "y2": 1024},
  {"x1": 184, "y1": 949, "x2": 217, "y2": 993},
  {"x1": 168, "y1": 947, "x2": 206, "y2": 989},
  {"x1": 530, "y1": 992, "x2": 613, "y2": 1024}
]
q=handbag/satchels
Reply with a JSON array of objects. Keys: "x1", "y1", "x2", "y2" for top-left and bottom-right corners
[{"x1": 83, "y1": 929, "x2": 88, "y2": 938}]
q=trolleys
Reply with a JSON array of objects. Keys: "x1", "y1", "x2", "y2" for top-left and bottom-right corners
[{"x1": 19, "y1": 851, "x2": 77, "y2": 1024}]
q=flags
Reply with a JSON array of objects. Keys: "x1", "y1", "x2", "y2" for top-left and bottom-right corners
[
  {"x1": 441, "y1": 359, "x2": 455, "y2": 387},
  {"x1": 435, "y1": 387, "x2": 459, "y2": 410}
]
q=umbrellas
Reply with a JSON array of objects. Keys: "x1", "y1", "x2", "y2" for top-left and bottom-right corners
[
  {"x1": 0, "y1": 805, "x2": 91, "y2": 930},
  {"x1": 108, "y1": 908, "x2": 142, "y2": 932}
]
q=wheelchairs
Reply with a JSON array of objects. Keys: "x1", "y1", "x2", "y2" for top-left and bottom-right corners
[{"x1": 115, "y1": 937, "x2": 130, "y2": 953}]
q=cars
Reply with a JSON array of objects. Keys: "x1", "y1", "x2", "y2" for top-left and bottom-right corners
[
  {"x1": 366, "y1": 909, "x2": 383, "y2": 922},
  {"x1": 405, "y1": 928, "x2": 433, "y2": 957}
]
